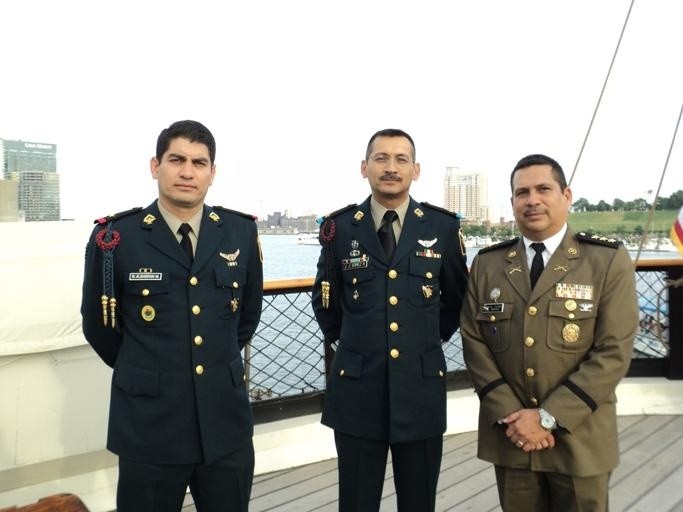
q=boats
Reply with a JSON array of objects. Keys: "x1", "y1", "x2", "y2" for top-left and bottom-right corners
[
  {"x1": 298, "y1": 234, "x2": 320, "y2": 245},
  {"x1": 462, "y1": 235, "x2": 501, "y2": 248},
  {"x1": 627, "y1": 236, "x2": 677, "y2": 251},
  {"x1": 631, "y1": 295, "x2": 669, "y2": 358}
]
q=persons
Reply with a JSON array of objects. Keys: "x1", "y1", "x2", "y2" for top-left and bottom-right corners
[
  {"x1": 459, "y1": 154, "x2": 638, "y2": 512},
  {"x1": 81, "y1": 120, "x2": 263, "y2": 511},
  {"x1": 312, "y1": 129, "x2": 470, "y2": 512}
]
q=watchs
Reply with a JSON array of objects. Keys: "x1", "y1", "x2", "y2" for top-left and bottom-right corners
[{"x1": 538, "y1": 407, "x2": 557, "y2": 431}]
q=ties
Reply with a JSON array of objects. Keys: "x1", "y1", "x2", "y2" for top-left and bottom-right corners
[
  {"x1": 178, "y1": 223, "x2": 194, "y2": 264},
  {"x1": 529, "y1": 243, "x2": 546, "y2": 289},
  {"x1": 377, "y1": 211, "x2": 397, "y2": 259}
]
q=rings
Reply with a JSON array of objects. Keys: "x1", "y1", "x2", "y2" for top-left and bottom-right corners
[{"x1": 518, "y1": 440, "x2": 524, "y2": 446}]
